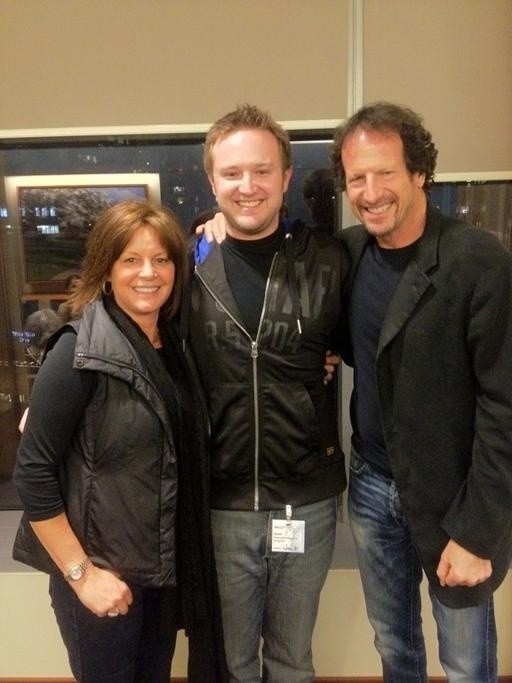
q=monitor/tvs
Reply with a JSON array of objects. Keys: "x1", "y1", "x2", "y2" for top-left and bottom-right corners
[{"x1": 4, "y1": 172, "x2": 161, "y2": 293}]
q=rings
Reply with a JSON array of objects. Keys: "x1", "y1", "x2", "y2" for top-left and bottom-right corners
[{"x1": 108, "y1": 608, "x2": 120, "y2": 617}]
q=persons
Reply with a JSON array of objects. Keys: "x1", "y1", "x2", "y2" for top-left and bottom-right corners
[
  {"x1": 301, "y1": 167, "x2": 341, "y2": 250},
  {"x1": 11, "y1": 197, "x2": 338, "y2": 682},
  {"x1": 64, "y1": 274, "x2": 83, "y2": 294},
  {"x1": 22, "y1": 303, "x2": 76, "y2": 374},
  {"x1": 196, "y1": 106, "x2": 512, "y2": 682},
  {"x1": 178, "y1": 106, "x2": 347, "y2": 683}
]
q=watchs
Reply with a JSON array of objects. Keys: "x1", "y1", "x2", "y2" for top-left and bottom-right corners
[{"x1": 65, "y1": 556, "x2": 90, "y2": 582}]
